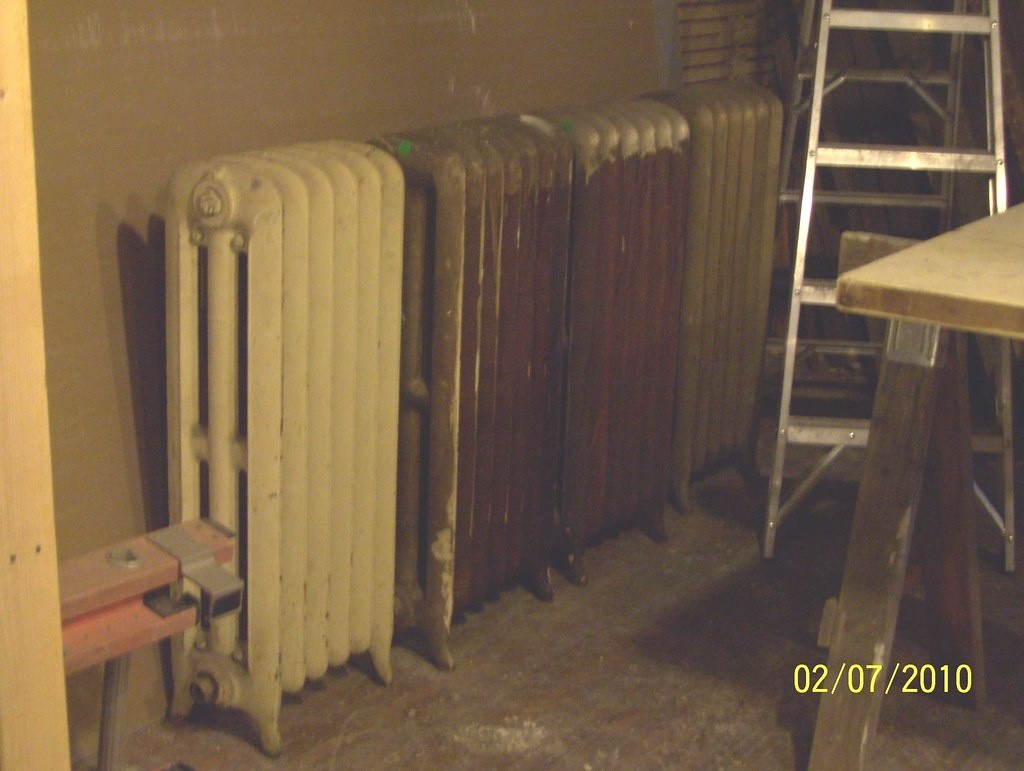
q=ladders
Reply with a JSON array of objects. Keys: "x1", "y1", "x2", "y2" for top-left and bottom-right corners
[{"x1": 747, "y1": 2, "x2": 1018, "y2": 574}]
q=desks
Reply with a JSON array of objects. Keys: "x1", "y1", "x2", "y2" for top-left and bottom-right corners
[{"x1": 836, "y1": 198, "x2": 1023, "y2": 342}]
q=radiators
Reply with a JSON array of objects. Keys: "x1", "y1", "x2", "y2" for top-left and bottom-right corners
[
  {"x1": 164, "y1": 137, "x2": 406, "y2": 759},
  {"x1": 365, "y1": 112, "x2": 577, "y2": 672},
  {"x1": 651, "y1": 78, "x2": 786, "y2": 518},
  {"x1": 519, "y1": 95, "x2": 692, "y2": 589}
]
